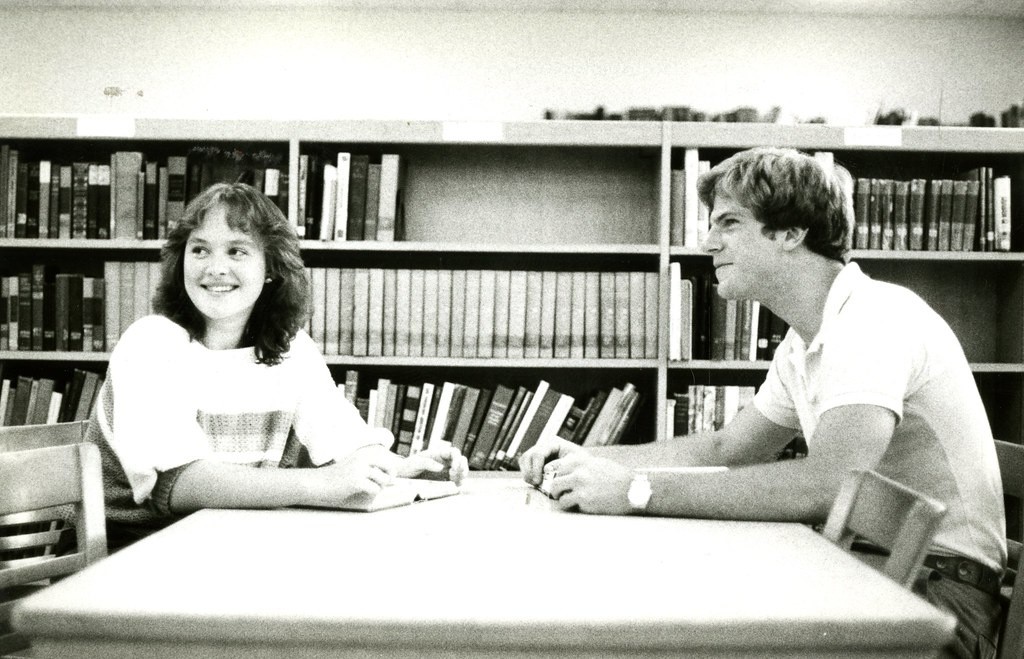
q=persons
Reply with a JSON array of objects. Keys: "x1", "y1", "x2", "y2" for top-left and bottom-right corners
[
  {"x1": 517, "y1": 142, "x2": 1010, "y2": 659},
  {"x1": 49, "y1": 182, "x2": 468, "y2": 587}
]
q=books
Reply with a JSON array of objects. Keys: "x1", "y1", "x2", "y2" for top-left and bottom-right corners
[
  {"x1": 0, "y1": 142, "x2": 1023, "y2": 474},
  {"x1": 285, "y1": 478, "x2": 460, "y2": 512}
]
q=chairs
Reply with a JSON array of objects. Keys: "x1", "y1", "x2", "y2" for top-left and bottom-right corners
[
  {"x1": 822, "y1": 466, "x2": 950, "y2": 591},
  {"x1": 992, "y1": 437, "x2": 1024, "y2": 659},
  {"x1": 0, "y1": 443, "x2": 109, "y2": 659}
]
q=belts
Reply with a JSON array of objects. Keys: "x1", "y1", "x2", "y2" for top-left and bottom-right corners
[{"x1": 850, "y1": 531, "x2": 1002, "y2": 597}]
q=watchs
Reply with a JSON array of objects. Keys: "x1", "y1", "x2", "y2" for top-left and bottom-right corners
[{"x1": 628, "y1": 468, "x2": 653, "y2": 515}]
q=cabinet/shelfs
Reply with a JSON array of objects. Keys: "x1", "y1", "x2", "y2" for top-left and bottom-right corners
[{"x1": 0, "y1": 116, "x2": 1024, "y2": 443}]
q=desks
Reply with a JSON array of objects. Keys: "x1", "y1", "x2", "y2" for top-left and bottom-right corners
[{"x1": 11, "y1": 472, "x2": 958, "y2": 659}]
